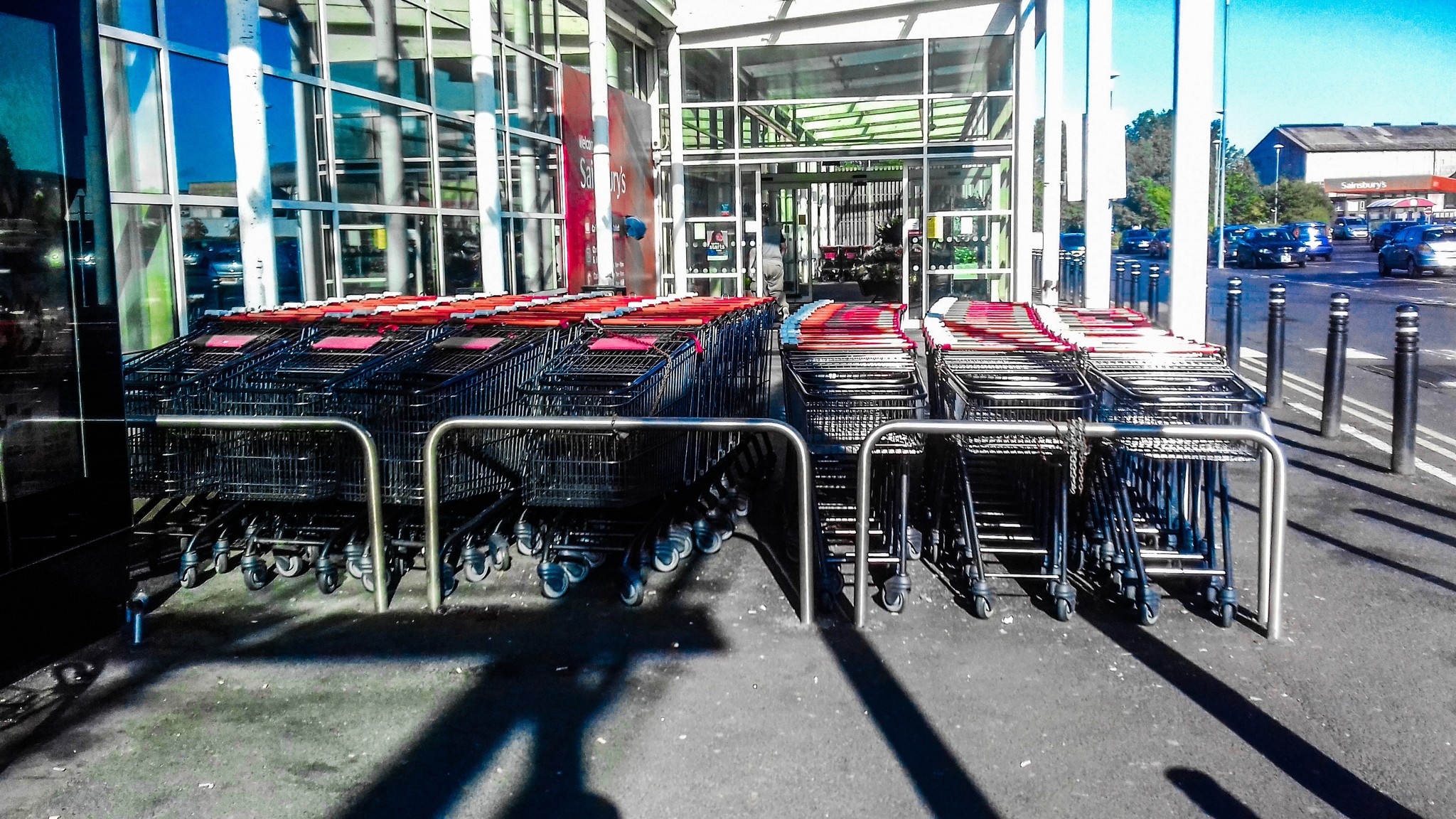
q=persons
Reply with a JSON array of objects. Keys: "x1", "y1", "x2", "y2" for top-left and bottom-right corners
[
  {"x1": 743, "y1": 211, "x2": 791, "y2": 325},
  {"x1": 707, "y1": 231, "x2": 727, "y2": 257}
]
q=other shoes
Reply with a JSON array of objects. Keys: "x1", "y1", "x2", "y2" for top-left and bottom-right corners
[{"x1": 781, "y1": 306, "x2": 790, "y2": 323}]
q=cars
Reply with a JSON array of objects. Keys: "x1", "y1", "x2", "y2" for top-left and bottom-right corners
[
  {"x1": 1279, "y1": 223, "x2": 1334, "y2": 262},
  {"x1": 1235, "y1": 228, "x2": 1309, "y2": 268},
  {"x1": 1149, "y1": 229, "x2": 1170, "y2": 257},
  {"x1": 1367, "y1": 221, "x2": 1421, "y2": 252},
  {"x1": 1118, "y1": 228, "x2": 1156, "y2": 254},
  {"x1": 1059, "y1": 233, "x2": 1085, "y2": 266},
  {"x1": 1378, "y1": 225, "x2": 1456, "y2": 278},
  {"x1": 1207, "y1": 225, "x2": 1258, "y2": 263},
  {"x1": 1333, "y1": 216, "x2": 1370, "y2": 239}
]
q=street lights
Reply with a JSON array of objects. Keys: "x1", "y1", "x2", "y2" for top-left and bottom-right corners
[
  {"x1": 1212, "y1": 140, "x2": 1222, "y2": 234},
  {"x1": 1274, "y1": 143, "x2": 1284, "y2": 225}
]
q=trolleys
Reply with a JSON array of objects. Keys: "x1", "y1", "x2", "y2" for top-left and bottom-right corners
[{"x1": 106, "y1": 283, "x2": 1269, "y2": 633}]
